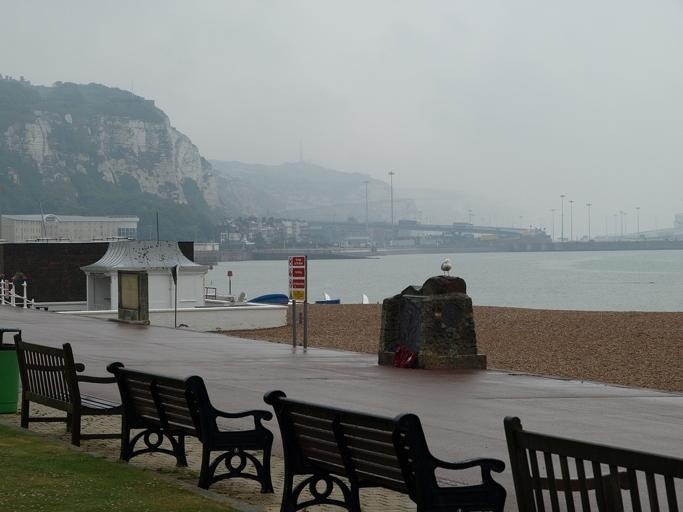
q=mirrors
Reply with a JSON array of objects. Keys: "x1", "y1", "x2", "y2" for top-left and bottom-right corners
[{"x1": 440, "y1": 258, "x2": 451, "y2": 278}]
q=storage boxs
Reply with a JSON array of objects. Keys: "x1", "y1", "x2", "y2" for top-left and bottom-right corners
[
  {"x1": 106, "y1": 361, "x2": 275, "y2": 493},
  {"x1": 14, "y1": 334, "x2": 130, "y2": 451},
  {"x1": 504, "y1": 416, "x2": 683, "y2": 512},
  {"x1": 263, "y1": 389, "x2": 507, "y2": 512}
]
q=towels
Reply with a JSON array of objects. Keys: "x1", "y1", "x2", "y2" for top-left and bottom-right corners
[{"x1": 0, "y1": 327, "x2": 22, "y2": 415}]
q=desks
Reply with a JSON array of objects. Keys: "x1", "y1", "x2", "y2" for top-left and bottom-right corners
[
  {"x1": 551, "y1": 194, "x2": 641, "y2": 242},
  {"x1": 388, "y1": 171, "x2": 396, "y2": 226},
  {"x1": 363, "y1": 180, "x2": 371, "y2": 237}
]
